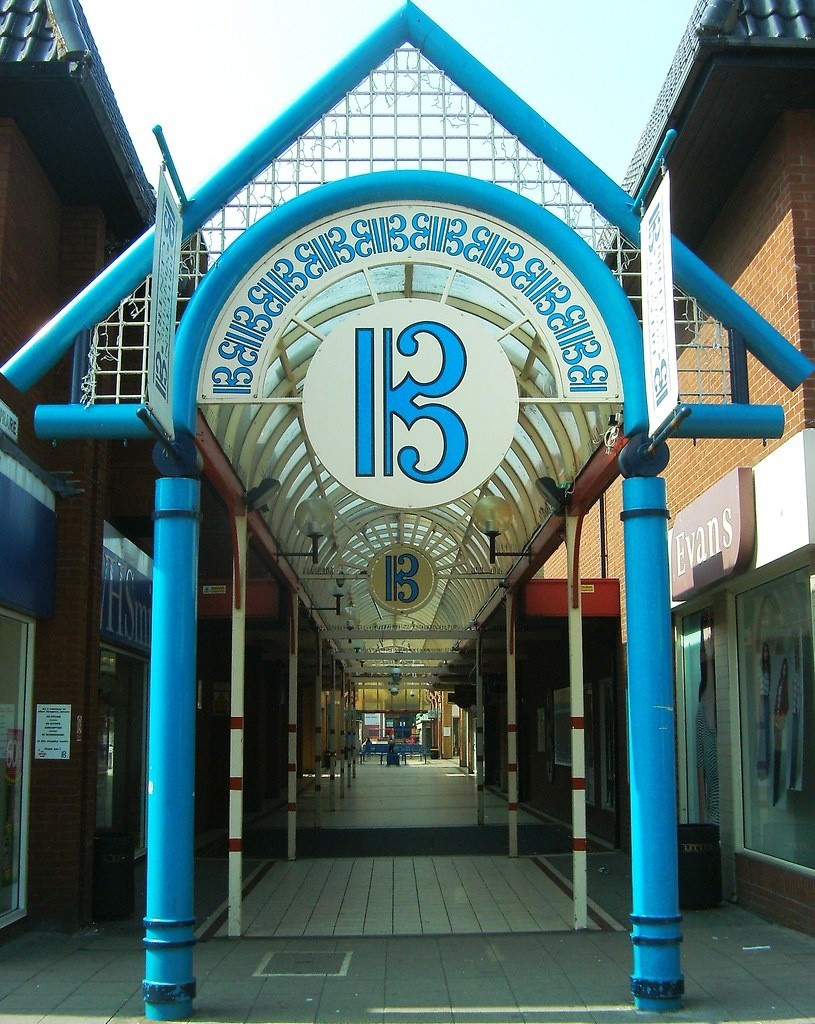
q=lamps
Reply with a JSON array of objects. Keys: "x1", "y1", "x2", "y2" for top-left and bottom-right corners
[
  {"x1": 409, "y1": 688, "x2": 415, "y2": 697},
  {"x1": 390, "y1": 667, "x2": 401, "y2": 696},
  {"x1": 276, "y1": 496, "x2": 335, "y2": 564},
  {"x1": 473, "y1": 495, "x2": 532, "y2": 563},
  {"x1": 310, "y1": 569, "x2": 366, "y2": 653}
]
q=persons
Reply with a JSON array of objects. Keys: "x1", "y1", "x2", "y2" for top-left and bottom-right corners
[
  {"x1": 695, "y1": 607, "x2": 720, "y2": 827},
  {"x1": 388, "y1": 738, "x2": 395, "y2": 753},
  {"x1": 790, "y1": 643, "x2": 802, "y2": 790},
  {"x1": 756, "y1": 643, "x2": 771, "y2": 772},
  {"x1": 773, "y1": 658, "x2": 788, "y2": 806},
  {"x1": 366, "y1": 738, "x2": 372, "y2": 744}
]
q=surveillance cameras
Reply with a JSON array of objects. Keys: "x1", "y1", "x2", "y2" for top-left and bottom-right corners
[
  {"x1": 391, "y1": 687, "x2": 398, "y2": 695},
  {"x1": 410, "y1": 691, "x2": 415, "y2": 696}
]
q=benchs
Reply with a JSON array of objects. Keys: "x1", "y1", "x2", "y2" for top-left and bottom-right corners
[{"x1": 357, "y1": 744, "x2": 432, "y2": 765}]
